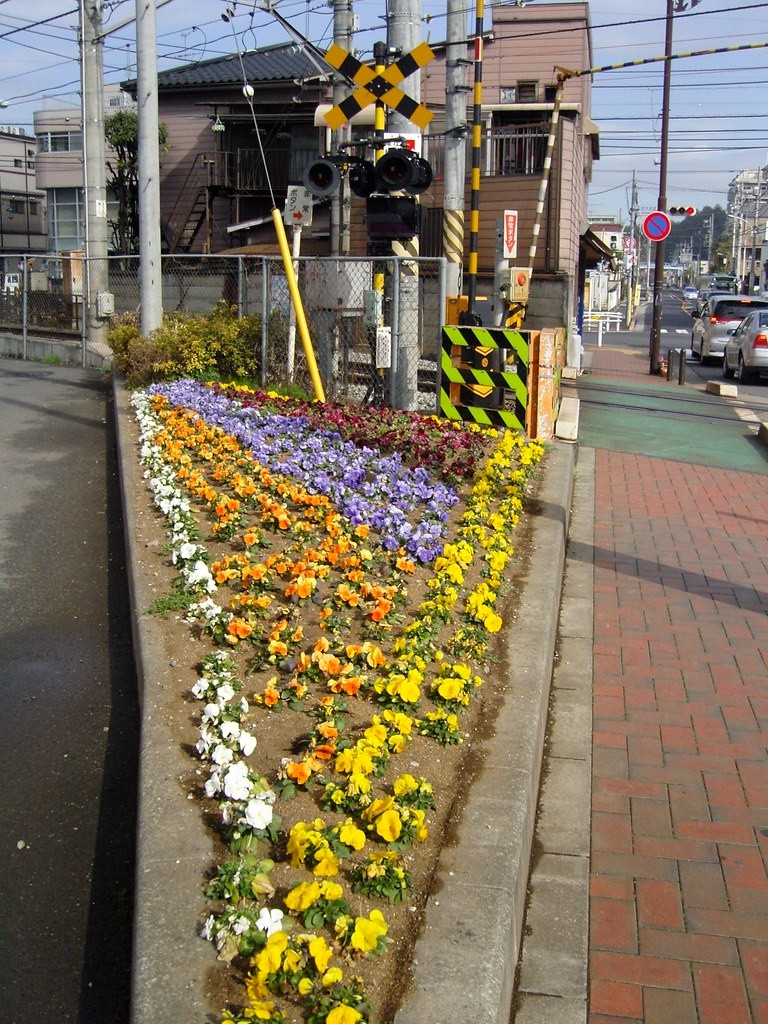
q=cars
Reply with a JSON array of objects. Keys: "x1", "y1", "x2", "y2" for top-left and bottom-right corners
[
  {"x1": 691, "y1": 295, "x2": 768, "y2": 367},
  {"x1": 722, "y1": 310, "x2": 768, "y2": 385},
  {"x1": 695, "y1": 291, "x2": 734, "y2": 314},
  {"x1": 683, "y1": 287, "x2": 699, "y2": 300},
  {"x1": 0, "y1": 273, "x2": 19, "y2": 295}
]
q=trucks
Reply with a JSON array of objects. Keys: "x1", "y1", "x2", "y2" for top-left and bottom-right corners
[{"x1": 700, "y1": 273, "x2": 738, "y2": 291}]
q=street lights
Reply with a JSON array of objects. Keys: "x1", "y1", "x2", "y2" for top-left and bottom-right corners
[{"x1": 728, "y1": 215, "x2": 757, "y2": 295}]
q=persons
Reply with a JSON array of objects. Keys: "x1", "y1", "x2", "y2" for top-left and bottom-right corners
[{"x1": 739, "y1": 276, "x2": 745, "y2": 294}]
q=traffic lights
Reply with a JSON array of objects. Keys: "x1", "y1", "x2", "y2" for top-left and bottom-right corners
[
  {"x1": 668, "y1": 207, "x2": 696, "y2": 216},
  {"x1": 305, "y1": 159, "x2": 340, "y2": 197},
  {"x1": 375, "y1": 153, "x2": 413, "y2": 191},
  {"x1": 367, "y1": 194, "x2": 416, "y2": 240}
]
